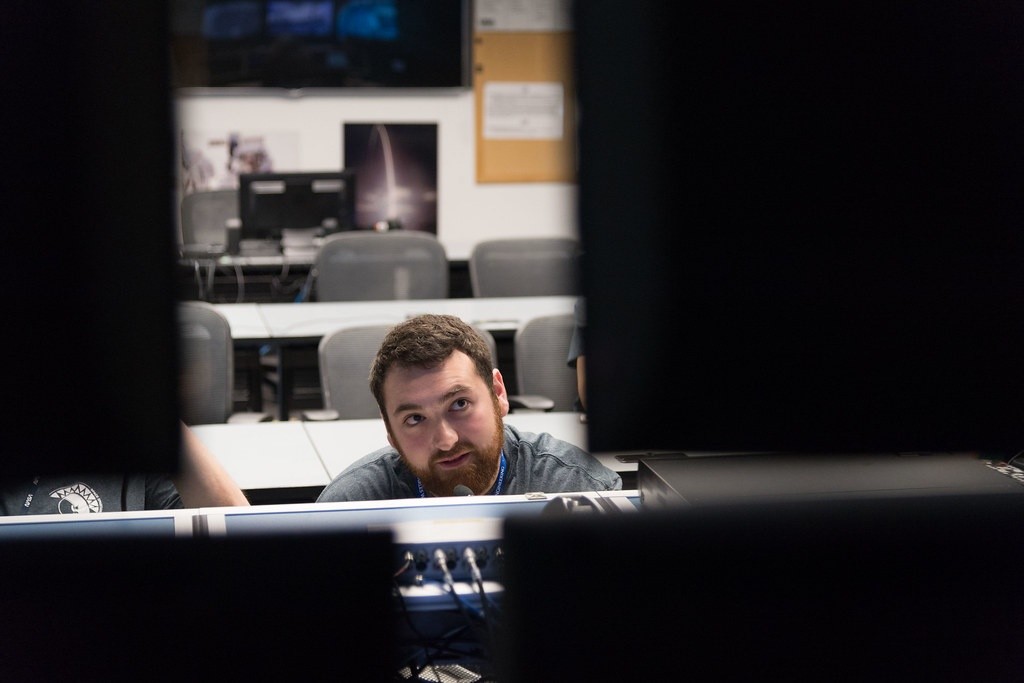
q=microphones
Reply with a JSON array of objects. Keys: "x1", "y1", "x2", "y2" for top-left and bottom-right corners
[{"x1": 453, "y1": 485, "x2": 474, "y2": 497}]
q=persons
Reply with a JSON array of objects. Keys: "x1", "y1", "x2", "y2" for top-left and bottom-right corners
[
  {"x1": 1, "y1": 415, "x2": 250, "y2": 514},
  {"x1": 314, "y1": 314, "x2": 622, "y2": 579},
  {"x1": 567, "y1": 297, "x2": 589, "y2": 412}
]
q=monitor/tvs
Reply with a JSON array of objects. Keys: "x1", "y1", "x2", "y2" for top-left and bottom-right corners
[{"x1": 239, "y1": 173, "x2": 356, "y2": 250}]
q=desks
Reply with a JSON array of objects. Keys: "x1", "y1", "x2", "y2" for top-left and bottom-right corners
[
  {"x1": 256, "y1": 295, "x2": 583, "y2": 422},
  {"x1": 213, "y1": 294, "x2": 272, "y2": 413},
  {"x1": 190, "y1": 413, "x2": 333, "y2": 505},
  {"x1": 302, "y1": 411, "x2": 739, "y2": 480},
  {"x1": 180, "y1": 248, "x2": 470, "y2": 279}
]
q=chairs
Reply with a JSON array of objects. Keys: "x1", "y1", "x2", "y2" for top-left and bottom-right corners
[
  {"x1": 317, "y1": 324, "x2": 497, "y2": 421},
  {"x1": 179, "y1": 187, "x2": 277, "y2": 414},
  {"x1": 469, "y1": 236, "x2": 581, "y2": 299},
  {"x1": 178, "y1": 298, "x2": 234, "y2": 426},
  {"x1": 314, "y1": 231, "x2": 451, "y2": 301},
  {"x1": 515, "y1": 314, "x2": 580, "y2": 413}
]
